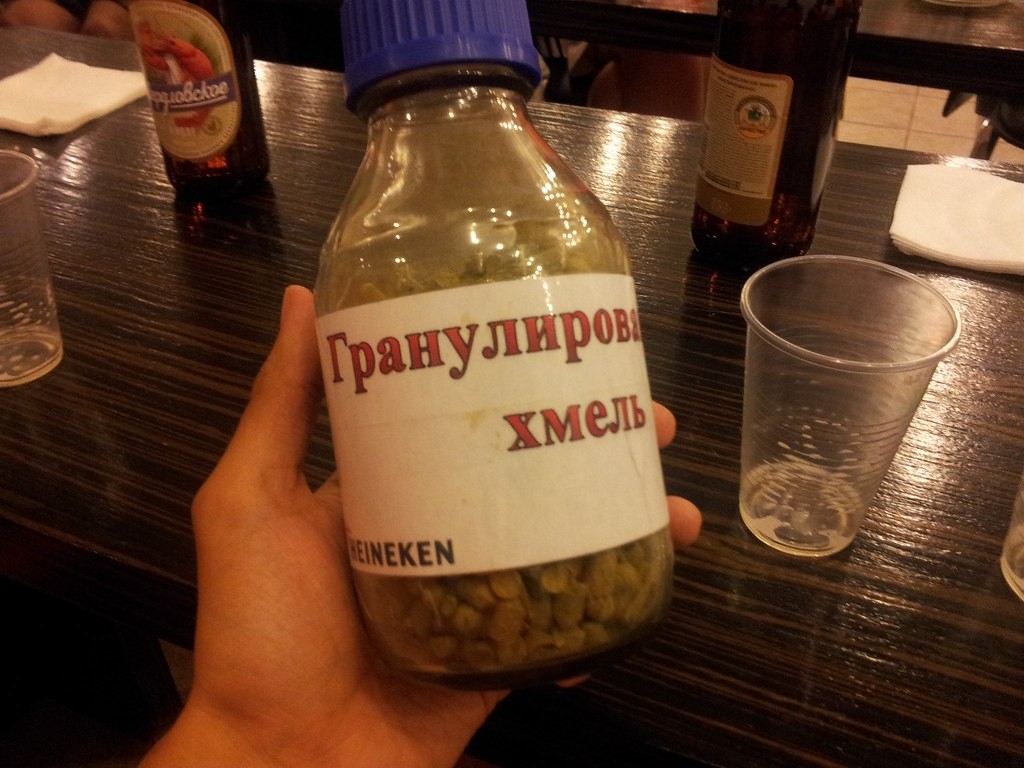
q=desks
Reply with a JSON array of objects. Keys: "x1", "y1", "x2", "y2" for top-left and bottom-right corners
[
  {"x1": 251, "y1": 0, "x2": 1024, "y2": 158},
  {"x1": 0, "y1": 21, "x2": 1024, "y2": 768}
]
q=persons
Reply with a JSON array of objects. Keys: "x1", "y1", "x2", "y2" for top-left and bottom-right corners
[{"x1": 126, "y1": 284, "x2": 701, "y2": 767}]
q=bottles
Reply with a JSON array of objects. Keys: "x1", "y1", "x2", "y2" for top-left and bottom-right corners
[
  {"x1": 690, "y1": 0, "x2": 863, "y2": 274},
  {"x1": 313, "y1": 0, "x2": 672, "y2": 683},
  {"x1": 129, "y1": 0, "x2": 269, "y2": 191}
]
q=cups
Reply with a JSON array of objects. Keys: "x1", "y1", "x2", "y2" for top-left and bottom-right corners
[
  {"x1": 0, "y1": 150, "x2": 63, "y2": 388},
  {"x1": 1001, "y1": 491, "x2": 1024, "y2": 601},
  {"x1": 739, "y1": 254, "x2": 961, "y2": 556}
]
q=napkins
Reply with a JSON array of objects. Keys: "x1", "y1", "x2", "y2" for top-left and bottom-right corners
[
  {"x1": 889, "y1": 163, "x2": 1024, "y2": 277},
  {"x1": 0, "y1": 51, "x2": 146, "y2": 137}
]
